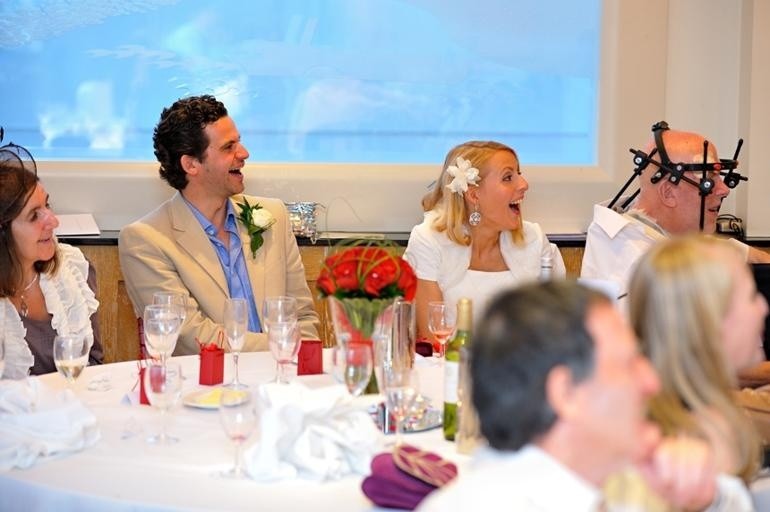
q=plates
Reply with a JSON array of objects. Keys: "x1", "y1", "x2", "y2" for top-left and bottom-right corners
[{"x1": 176, "y1": 383, "x2": 252, "y2": 408}]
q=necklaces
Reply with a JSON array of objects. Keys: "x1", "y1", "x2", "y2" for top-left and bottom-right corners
[{"x1": 12, "y1": 271, "x2": 40, "y2": 320}]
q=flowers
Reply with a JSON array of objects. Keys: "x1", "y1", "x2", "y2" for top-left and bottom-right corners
[{"x1": 316, "y1": 235, "x2": 418, "y2": 395}]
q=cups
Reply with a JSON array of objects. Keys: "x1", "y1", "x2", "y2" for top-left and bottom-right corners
[
  {"x1": 140, "y1": 287, "x2": 189, "y2": 360},
  {"x1": 335, "y1": 340, "x2": 374, "y2": 400},
  {"x1": 52, "y1": 333, "x2": 90, "y2": 386},
  {"x1": 372, "y1": 295, "x2": 423, "y2": 428}
]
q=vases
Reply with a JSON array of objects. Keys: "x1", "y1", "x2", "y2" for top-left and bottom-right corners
[{"x1": 327, "y1": 293, "x2": 414, "y2": 411}]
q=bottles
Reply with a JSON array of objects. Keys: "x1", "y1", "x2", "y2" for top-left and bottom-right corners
[{"x1": 442, "y1": 298, "x2": 474, "y2": 441}]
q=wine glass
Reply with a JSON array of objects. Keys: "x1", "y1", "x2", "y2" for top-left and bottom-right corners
[
  {"x1": 222, "y1": 296, "x2": 249, "y2": 394},
  {"x1": 217, "y1": 385, "x2": 261, "y2": 482},
  {"x1": 262, "y1": 294, "x2": 302, "y2": 386},
  {"x1": 428, "y1": 300, "x2": 458, "y2": 358}
]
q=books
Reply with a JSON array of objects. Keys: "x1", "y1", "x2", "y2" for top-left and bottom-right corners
[{"x1": 53, "y1": 214, "x2": 102, "y2": 239}]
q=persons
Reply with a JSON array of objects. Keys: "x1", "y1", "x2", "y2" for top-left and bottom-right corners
[
  {"x1": 119, "y1": 94, "x2": 324, "y2": 358},
  {"x1": 413, "y1": 278, "x2": 661, "y2": 511},
  {"x1": 0, "y1": 142, "x2": 105, "y2": 381},
  {"x1": 401, "y1": 139, "x2": 553, "y2": 352},
  {"x1": 628, "y1": 232, "x2": 770, "y2": 511},
  {"x1": 578, "y1": 121, "x2": 770, "y2": 329}
]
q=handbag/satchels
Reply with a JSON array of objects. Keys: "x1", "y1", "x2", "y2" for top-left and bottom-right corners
[{"x1": 360, "y1": 444, "x2": 458, "y2": 508}]
